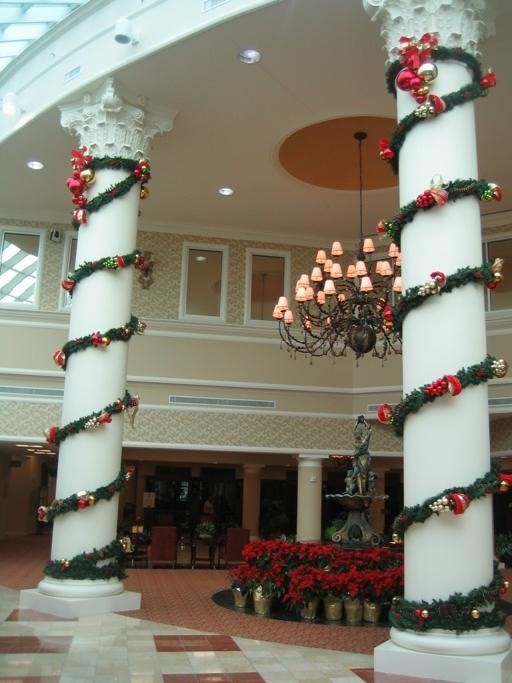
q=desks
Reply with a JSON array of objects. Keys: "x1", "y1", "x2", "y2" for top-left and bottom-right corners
[{"x1": 191, "y1": 536, "x2": 217, "y2": 569}]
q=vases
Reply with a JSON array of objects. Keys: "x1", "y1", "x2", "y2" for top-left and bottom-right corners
[{"x1": 300, "y1": 596, "x2": 380, "y2": 622}]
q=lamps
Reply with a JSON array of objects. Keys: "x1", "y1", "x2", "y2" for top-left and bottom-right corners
[
  {"x1": 2, "y1": 91, "x2": 26, "y2": 119},
  {"x1": 113, "y1": 18, "x2": 139, "y2": 48},
  {"x1": 272, "y1": 132, "x2": 401, "y2": 369}
]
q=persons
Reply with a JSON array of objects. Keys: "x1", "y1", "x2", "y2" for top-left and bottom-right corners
[{"x1": 341, "y1": 414, "x2": 377, "y2": 495}]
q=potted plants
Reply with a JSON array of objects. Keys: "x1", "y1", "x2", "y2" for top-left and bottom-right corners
[{"x1": 194, "y1": 520, "x2": 218, "y2": 538}]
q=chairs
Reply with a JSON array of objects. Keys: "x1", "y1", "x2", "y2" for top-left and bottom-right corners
[
  {"x1": 146, "y1": 526, "x2": 177, "y2": 569},
  {"x1": 218, "y1": 527, "x2": 249, "y2": 569}
]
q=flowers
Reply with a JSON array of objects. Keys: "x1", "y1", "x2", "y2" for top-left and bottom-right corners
[{"x1": 227, "y1": 538, "x2": 404, "y2": 615}]
